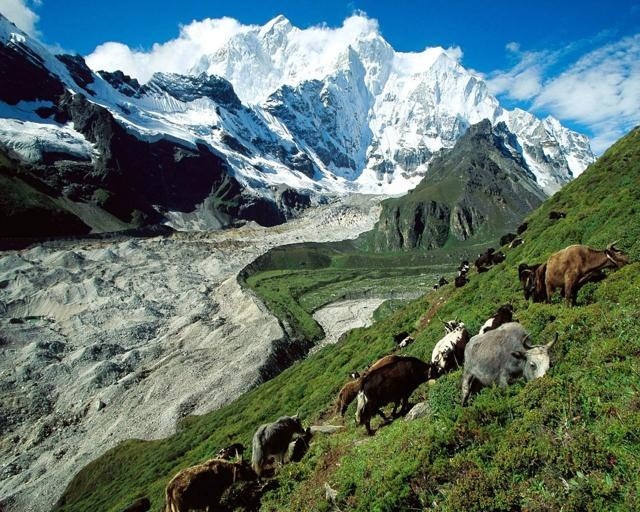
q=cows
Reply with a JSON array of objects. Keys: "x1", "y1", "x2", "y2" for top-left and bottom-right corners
[
  {"x1": 430, "y1": 303, "x2": 559, "y2": 407},
  {"x1": 215, "y1": 443, "x2": 246, "y2": 462},
  {"x1": 392, "y1": 331, "x2": 414, "y2": 348},
  {"x1": 454, "y1": 223, "x2": 530, "y2": 288},
  {"x1": 433, "y1": 276, "x2": 449, "y2": 291},
  {"x1": 517, "y1": 239, "x2": 632, "y2": 308},
  {"x1": 164, "y1": 457, "x2": 258, "y2": 512},
  {"x1": 337, "y1": 354, "x2": 446, "y2": 436},
  {"x1": 250, "y1": 412, "x2": 306, "y2": 479},
  {"x1": 549, "y1": 211, "x2": 566, "y2": 219}
]
